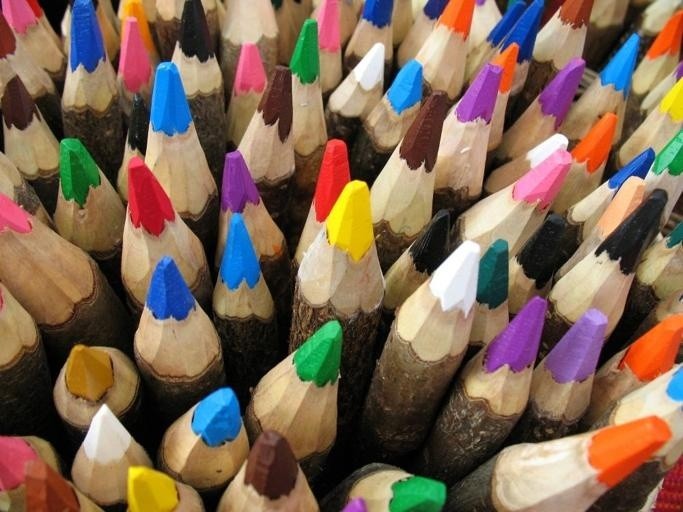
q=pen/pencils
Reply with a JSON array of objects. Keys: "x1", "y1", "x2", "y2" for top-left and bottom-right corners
[{"x1": 0, "y1": 0, "x2": 683, "y2": 512}]
